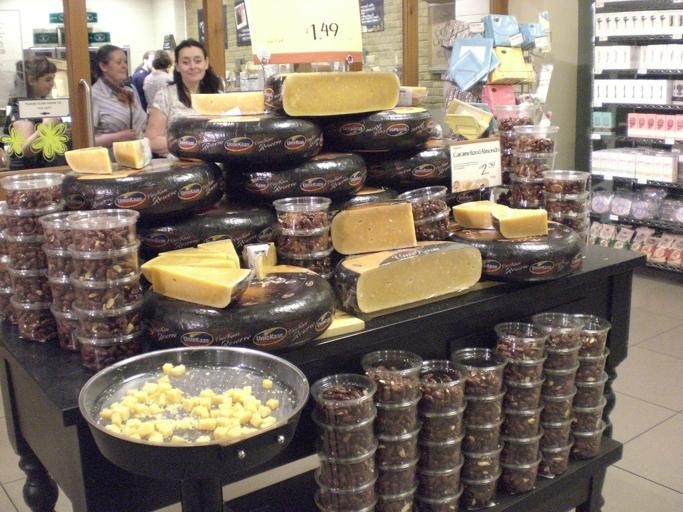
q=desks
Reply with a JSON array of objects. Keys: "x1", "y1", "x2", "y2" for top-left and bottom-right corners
[{"x1": 0, "y1": 241, "x2": 650, "y2": 512}]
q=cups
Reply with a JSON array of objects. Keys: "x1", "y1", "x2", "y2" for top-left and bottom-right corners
[
  {"x1": 269, "y1": 195, "x2": 335, "y2": 293},
  {"x1": 495, "y1": 104, "x2": 590, "y2": 247},
  {"x1": 305, "y1": 310, "x2": 613, "y2": 512},
  {"x1": 0, "y1": 169, "x2": 146, "y2": 371},
  {"x1": 398, "y1": 184, "x2": 451, "y2": 243}
]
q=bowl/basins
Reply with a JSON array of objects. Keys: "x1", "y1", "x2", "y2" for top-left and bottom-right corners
[{"x1": 80, "y1": 345, "x2": 311, "y2": 483}]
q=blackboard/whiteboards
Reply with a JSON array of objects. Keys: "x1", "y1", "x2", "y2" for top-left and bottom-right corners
[
  {"x1": 358, "y1": 1, "x2": 385, "y2": 33},
  {"x1": 198, "y1": 0, "x2": 249, "y2": 49}
]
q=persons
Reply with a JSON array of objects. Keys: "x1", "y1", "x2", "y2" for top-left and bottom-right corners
[
  {"x1": 132, "y1": 50, "x2": 154, "y2": 111},
  {"x1": 0, "y1": 56, "x2": 64, "y2": 169},
  {"x1": 143, "y1": 49, "x2": 174, "y2": 107},
  {"x1": 91, "y1": 44, "x2": 148, "y2": 162},
  {"x1": 145, "y1": 38, "x2": 228, "y2": 158}
]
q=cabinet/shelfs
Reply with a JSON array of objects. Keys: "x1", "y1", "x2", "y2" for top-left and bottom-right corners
[{"x1": 576, "y1": 1, "x2": 683, "y2": 273}]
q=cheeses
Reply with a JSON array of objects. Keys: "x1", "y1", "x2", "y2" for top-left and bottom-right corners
[{"x1": 64, "y1": 70, "x2": 547, "y2": 316}]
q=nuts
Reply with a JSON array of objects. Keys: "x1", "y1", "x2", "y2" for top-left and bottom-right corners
[
  {"x1": 0, "y1": 184, "x2": 143, "y2": 372},
  {"x1": 312, "y1": 320, "x2": 608, "y2": 512}
]
